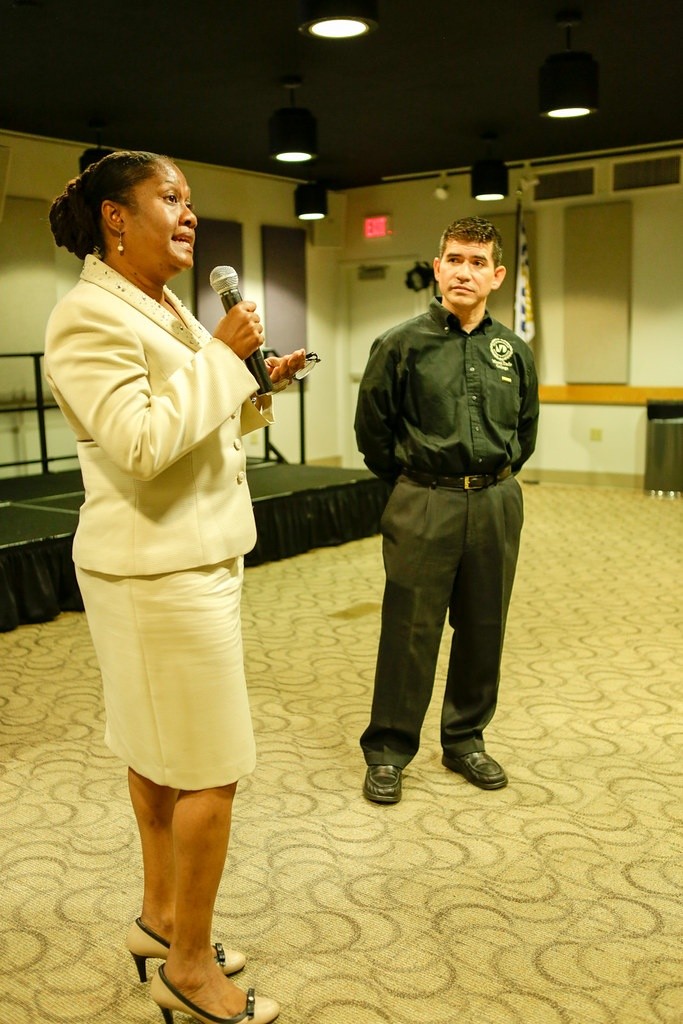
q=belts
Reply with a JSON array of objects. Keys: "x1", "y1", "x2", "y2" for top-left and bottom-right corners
[{"x1": 403, "y1": 465, "x2": 511, "y2": 489}]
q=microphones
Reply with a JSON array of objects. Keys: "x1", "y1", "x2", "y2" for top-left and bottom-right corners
[{"x1": 209, "y1": 265, "x2": 274, "y2": 397}]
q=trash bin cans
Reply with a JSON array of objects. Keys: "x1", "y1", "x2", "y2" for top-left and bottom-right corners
[{"x1": 643, "y1": 398, "x2": 683, "y2": 499}]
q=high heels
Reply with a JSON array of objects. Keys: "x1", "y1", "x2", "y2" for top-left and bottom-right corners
[
  {"x1": 150, "y1": 963, "x2": 278, "y2": 1023},
  {"x1": 127, "y1": 916, "x2": 246, "y2": 982}
]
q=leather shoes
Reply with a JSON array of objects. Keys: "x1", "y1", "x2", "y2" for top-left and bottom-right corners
[
  {"x1": 441, "y1": 751, "x2": 508, "y2": 789},
  {"x1": 362, "y1": 765, "x2": 401, "y2": 801}
]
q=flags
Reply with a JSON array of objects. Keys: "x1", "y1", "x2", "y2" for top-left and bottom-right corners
[{"x1": 511, "y1": 186, "x2": 536, "y2": 359}]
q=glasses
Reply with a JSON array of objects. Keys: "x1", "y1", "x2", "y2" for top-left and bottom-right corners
[{"x1": 254, "y1": 352, "x2": 320, "y2": 398}]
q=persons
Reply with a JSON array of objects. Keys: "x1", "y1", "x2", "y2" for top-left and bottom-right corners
[
  {"x1": 352, "y1": 217, "x2": 540, "y2": 807},
  {"x1": 41, "y1": 150, "x2": 306, "y2": 1024}
]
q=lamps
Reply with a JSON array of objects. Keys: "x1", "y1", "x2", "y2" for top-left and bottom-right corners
[
  {"x1": 269, "y1": 76, "x2": 320, "y2": 163},
  {"x1": 469, "y1": 135, "x2": 510, "y2": 202},
  {"x1": 292, "y1": 170, "x2": 329, "y2": 222},
  {"x1": 537, "y1": 10, "x2": 603, "y2": 122},
  {"x1": 296, "y1": 0, "x2": 380, "y2": 41}
]
